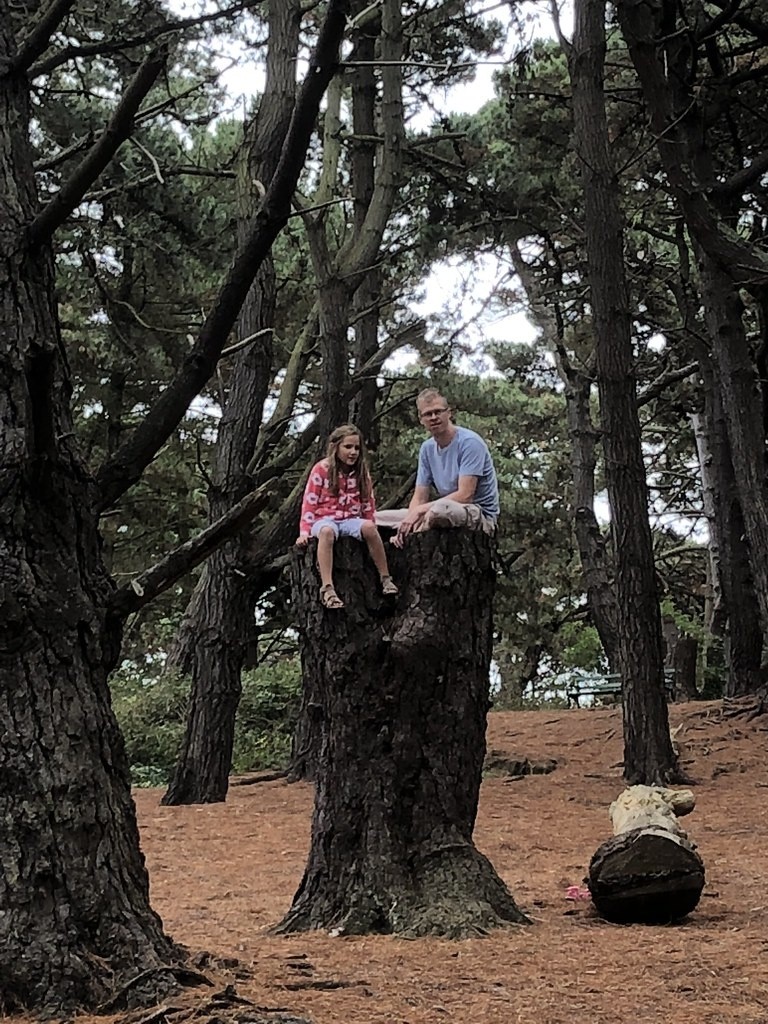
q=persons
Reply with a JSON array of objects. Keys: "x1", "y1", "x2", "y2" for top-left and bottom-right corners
[
  {"x1": 296, "y1": 424, "x2": 399, "y2": 608},
  {"x1": 373, "y1": 389, "x2": 501, "y2": 550}
]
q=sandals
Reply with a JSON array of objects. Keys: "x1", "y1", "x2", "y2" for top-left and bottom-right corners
[
  {"x1": 319, "y1": 584, "x2": 345, "y2": 609},
  {"x1": 381, "y1": 575, "x2": 398, "y2": 594}
]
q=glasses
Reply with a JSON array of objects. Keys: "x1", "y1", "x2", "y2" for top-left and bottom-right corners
[{"x1": 420, "y1": 407, "x2": 449, "y2": 420}]
